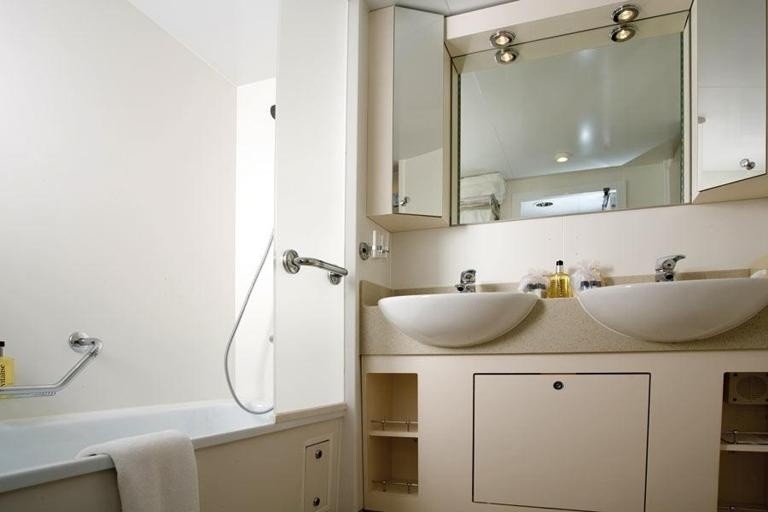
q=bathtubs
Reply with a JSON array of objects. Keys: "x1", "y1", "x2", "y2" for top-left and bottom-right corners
[{"x1": 1, "y1": 398, "x2": 275, "y2": 496}]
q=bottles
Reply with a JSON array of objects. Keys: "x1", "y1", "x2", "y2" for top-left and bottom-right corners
[
  {"x1": 578, "y1": 279, "x2": 602, "y2": 293},
  {"x1": 0, "y1": 340, "x2": 15, "y2": 390},
  {"x1": 523, "y1": 282, "x2": 546, "y2": 299}
]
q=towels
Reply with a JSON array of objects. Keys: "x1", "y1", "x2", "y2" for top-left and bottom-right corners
[{"x1": 76, "y1": 427, "x2": 202, "y2": 510}]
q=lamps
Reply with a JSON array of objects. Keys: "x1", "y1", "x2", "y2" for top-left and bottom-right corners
[
  {"x1": 610, "y1": 5, "x2": 639, "y2": 23},
  {"x1": 494, "y1": 48, "x2": 517, "y2": 63},
  {"x1": 486, "y1": 29, "x2": 516, "y2": 47},
  {"x1": 607, "y1": 26, "x2": 638, "y2": 43}
]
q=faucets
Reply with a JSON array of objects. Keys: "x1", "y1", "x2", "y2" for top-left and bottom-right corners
[
  {"x1": 454, "y1": 268, "x2": 478, "y2": 292},
  {"x1": 654, "y1": 253, "x2": 686, "y2": 282}
]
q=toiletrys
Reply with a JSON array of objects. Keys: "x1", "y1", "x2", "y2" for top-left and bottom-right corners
[{"x1": 1, "y1": 342, "x2": 16, "y2": 387}]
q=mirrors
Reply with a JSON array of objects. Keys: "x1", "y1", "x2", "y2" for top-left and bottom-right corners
[{"x1": 451, "y1": 11, "x2": 692, "y2": 227}]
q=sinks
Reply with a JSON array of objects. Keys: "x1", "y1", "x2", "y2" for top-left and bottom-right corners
[
  {"x1": 377, "y1": 291, "x2": 540, "y2": 350},
  {"x1": 578, "y1": 275, "x2": 768, "y2": 346}
]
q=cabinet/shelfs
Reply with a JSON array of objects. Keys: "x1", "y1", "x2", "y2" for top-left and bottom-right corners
[
  {"x1": 360, "y1": 349, "x2": 765, "y2": 511},
  {"x1": 692, "y1": 1, "x2": 767, "y2": 205},
  {"x1": 367, "y1": 6, "x2": 452, "y2": 231}
]
